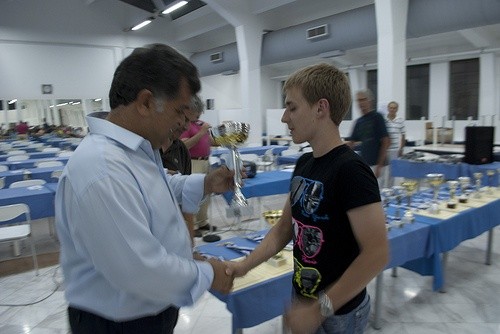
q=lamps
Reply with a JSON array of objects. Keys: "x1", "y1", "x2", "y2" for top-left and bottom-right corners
[
  {"x1": 158, "y1": 0, "x2": 188, "y2": 17},
  {"x1": 123, "y1": 16, "x2": 156, "y2": 32}
]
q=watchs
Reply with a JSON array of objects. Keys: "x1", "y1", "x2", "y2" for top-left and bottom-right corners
[{"x1": 318, "y1": 291, "x2": 334, "y2": 317}]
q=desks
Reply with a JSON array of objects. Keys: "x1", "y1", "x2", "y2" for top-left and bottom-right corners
[
  {"x1": 0, "y1": 131, "x2": 82, "y2": 218},
  {"x1": 192, "y1": 143, "x2": 500, "y2": 334}
]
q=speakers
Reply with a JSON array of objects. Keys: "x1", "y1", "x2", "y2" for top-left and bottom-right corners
[{"x1": 464, "y1": 126, "x2": 494, "y2": 163}]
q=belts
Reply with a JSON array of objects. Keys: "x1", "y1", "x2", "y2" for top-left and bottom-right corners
[{"x1": 190, "y1": 156, "x2": 209, "y2": 160}]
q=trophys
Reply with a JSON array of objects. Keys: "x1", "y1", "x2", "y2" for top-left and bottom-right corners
[
  {"x1": 210, "y1": 119, "x2": 253, "y2": 208},
  {"x1": 264, "y1": 209, "x2": 287, "y2": 266},
  {"x1": 382, "y1": 169, "x2": 500, "y2": 231}
]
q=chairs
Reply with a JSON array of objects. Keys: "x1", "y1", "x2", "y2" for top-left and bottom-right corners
[
  {"x1": 0, "y1": 137, "x2": 83, "y2": 278},
  {"x1": 209, "y1": 141, "x2": 313, "y2": 170}
]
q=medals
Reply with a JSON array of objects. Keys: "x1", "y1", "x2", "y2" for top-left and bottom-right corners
[
  {"x1": 217, "y1": 241, "x2": 226, "y2": 247},
  {"x1": 242, "y1": 235, "x2": 253, "y2": 239}
]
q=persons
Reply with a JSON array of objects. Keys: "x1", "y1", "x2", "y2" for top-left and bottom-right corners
[
  {"x1": 179, "y1": 94, "x2": 218, "y2": 238},
  {"x1": 346, "y1": 89, "x2": 389, "y2": 189},
  {"x1": 219, "y1": 63, "x2": 391, "y2": 334},
  {"x1": 158, "y1": 135, "x2": 196, "y2": 251},
  {"x1": 54, "y1": 44, "x2": 234, "y2": 334},
  {"x1": 384, "y1": 102, "x2": 407, "y2": 159},
  {"x1": 0, "y1": 118, "x2": 82, "y2": 142}
]
